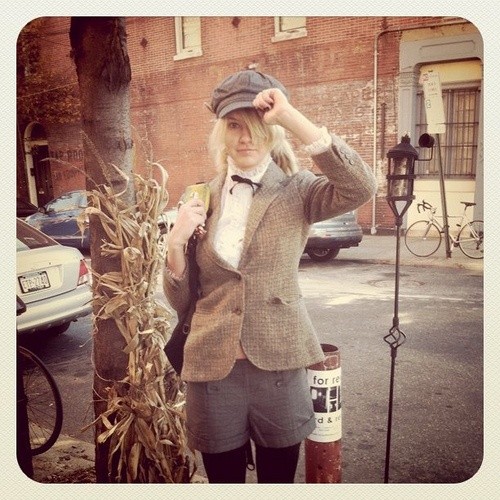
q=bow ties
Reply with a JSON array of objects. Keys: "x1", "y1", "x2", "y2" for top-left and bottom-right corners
[{"x1": 230, "y1": 174, "x2": 262, "y2": 197}]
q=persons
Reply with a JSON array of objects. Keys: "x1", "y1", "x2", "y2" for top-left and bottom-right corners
[{"x1": 161, "y1": 70, "x2": 378, "y2": 484}]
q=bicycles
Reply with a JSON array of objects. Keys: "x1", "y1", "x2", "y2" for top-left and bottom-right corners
[
  {"x1": 15, "y1": 296, "x2": 63, "y2": 457},
  {"x1": 404, "y1": 201, "x2": 484, "y2": 260}
]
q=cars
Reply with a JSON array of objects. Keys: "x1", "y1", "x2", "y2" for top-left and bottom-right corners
[
  {"x1": 19, "y1": 190, "x2": 90, "y2": 252},
  {"x1": 16, "y1": 218, "x2": 93, "y2": 336},
  {"x1": 148, "y1": 175, "x2": 363, "y2": 267}
]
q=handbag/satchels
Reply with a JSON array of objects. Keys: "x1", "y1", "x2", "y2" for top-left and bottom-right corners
[{"x1": 163, "y1": 307, "x2": 194, "y2": 375}]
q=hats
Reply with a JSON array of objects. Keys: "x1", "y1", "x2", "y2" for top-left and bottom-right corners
[{"x1": 205, "y1": 70, "x2": 288, "y2": 119}]
